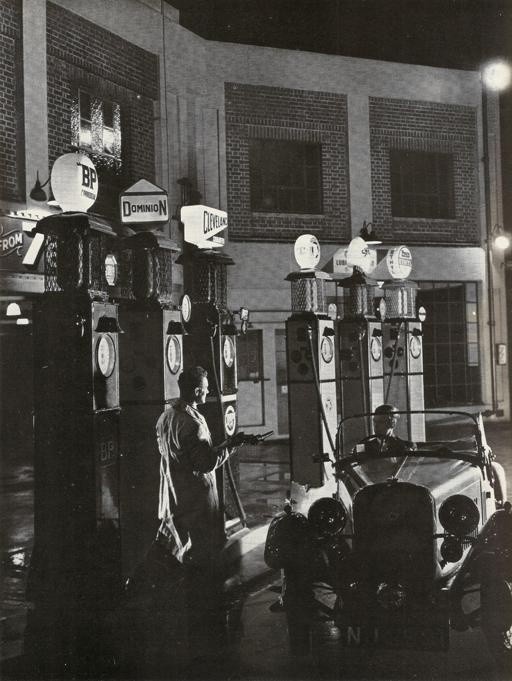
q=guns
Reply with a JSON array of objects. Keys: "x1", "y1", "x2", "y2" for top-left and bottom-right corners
[{"x1": 245, "y1": 431, "x2": 274, "y2": 445}]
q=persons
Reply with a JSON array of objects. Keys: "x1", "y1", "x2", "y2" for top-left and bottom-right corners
[
  {"x1": 150, "y1": 362, "x2": 261, "y2": 649},
  {"x1": 352, "y1": 404, "x2": 419, "y2": 464}
]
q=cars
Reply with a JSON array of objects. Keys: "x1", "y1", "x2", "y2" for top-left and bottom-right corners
[{"x1": 265, "y1": 410, "x2": 512, "y2": 670}]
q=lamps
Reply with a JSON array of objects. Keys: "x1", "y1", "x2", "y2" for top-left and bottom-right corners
[
  {"x1": 16, "y1": 318, "x2": 29, "y2": 325},
  {"x1": 30, "y1": 170, "x2": 58, "y2": 202},
  {"x1": 6, "y1": 302, "x2": 21, "y2": 316},
  {"x1": 483, "y1": 222, "x2": 509, "y2": 249}
]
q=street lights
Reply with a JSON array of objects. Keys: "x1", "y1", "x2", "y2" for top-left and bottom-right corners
[{"x1": 480, "y1": 60, "x2": 509, "y2": 416}]
